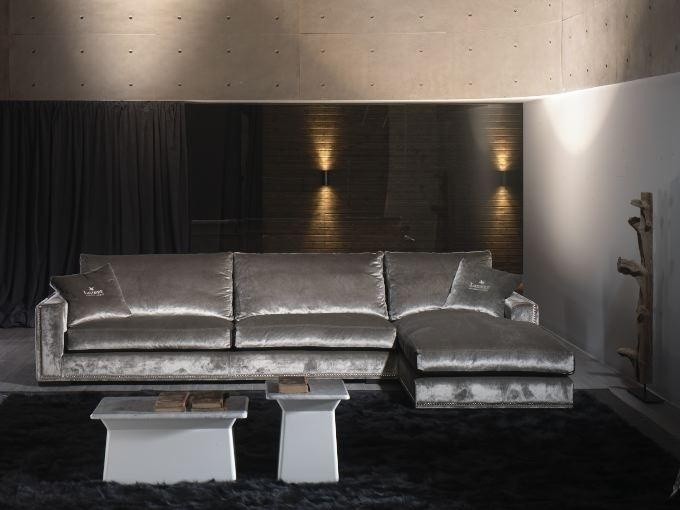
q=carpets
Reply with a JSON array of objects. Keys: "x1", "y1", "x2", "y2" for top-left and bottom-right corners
[{"x1": 0, "y1": 387, "x2": 680, "y2": 510}]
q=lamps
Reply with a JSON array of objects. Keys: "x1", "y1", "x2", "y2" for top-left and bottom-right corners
[
  {"x1": 495, "y1": 171, "x2": 512, "y2": 188},
  {"x1": 320, "y1": 170, "x2": 333, "y2": 186}
]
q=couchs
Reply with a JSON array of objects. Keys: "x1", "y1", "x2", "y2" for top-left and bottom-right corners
[{"x1": 33, "y1": 249, "x2": 574, "y2": 410}]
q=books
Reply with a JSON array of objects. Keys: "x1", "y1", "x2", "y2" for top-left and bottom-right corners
[
  {"x1": 154, "y1": 390, "x2": 229, "y2": 413},
  {"x1": 278, "y1": 374, "x2": 311, "y2": 395}
]
